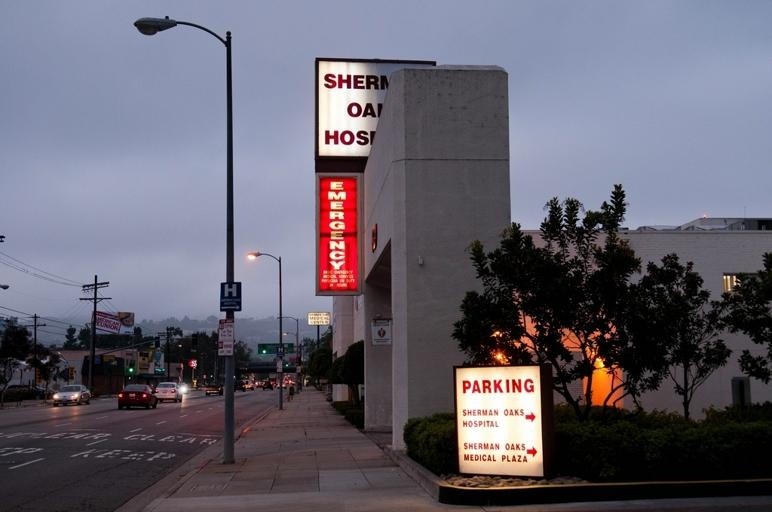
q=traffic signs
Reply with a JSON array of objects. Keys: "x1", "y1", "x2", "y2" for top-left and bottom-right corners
[{"x1": 257, "y1": 343, "x2": 294, "y2": 355}]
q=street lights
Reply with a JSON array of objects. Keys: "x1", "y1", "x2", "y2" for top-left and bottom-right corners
[
  {"x1": 276, "y1": 317, "x2": 302, "y2": 366},
  {"x1": 131, "y1": 16, "x2": 235, "y2": 464},
  {"x1": 248, "y1": 252, "x2": 285, "y2": 410}
]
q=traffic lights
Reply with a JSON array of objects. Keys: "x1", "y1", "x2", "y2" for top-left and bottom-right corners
[{"x1": 129, "y1": 368, "x2": 134, "y2": 372}]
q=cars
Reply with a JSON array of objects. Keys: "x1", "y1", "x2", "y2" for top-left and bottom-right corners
[
  {"x1": 6, "y1": 385, "x2": 92, "y2": 405},
  {"x1": 235, "y1": 380, "x2": 287, "y2": 392},
  {"x1": 117, "y1": 384, "x2": 157, "y2": 410},
  {"x1": 204, "y1": 384, "x2": 224, "y2": 396},
  {"x1": 156, "y1": 382, "x2": 182, "y2": 403}
]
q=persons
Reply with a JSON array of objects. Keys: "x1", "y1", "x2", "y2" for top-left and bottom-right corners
[
  {"x1": 273, "y1": 383, "x2": 277, "y2": 392},
  {"x1": 288, "y1": 385, "x2": 294, "y2": 401}
]
